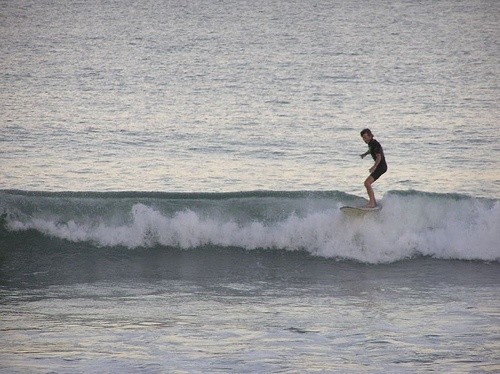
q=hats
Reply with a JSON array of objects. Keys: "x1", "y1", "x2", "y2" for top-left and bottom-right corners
[{"x1": 361, "y1": 128, "x2": 374, "y2": 137}]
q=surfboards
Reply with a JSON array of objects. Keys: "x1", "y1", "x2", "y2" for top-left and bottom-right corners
[{"x1": 339, "y1": 202, "x2": 382, "y2": 217}]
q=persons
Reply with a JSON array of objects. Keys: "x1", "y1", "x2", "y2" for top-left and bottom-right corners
[{"x1": 359, "y1": 128, "x2": 387, "y2": 208}]
